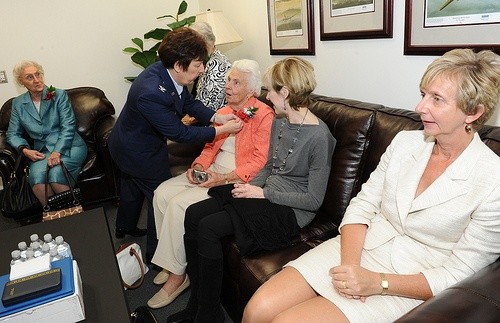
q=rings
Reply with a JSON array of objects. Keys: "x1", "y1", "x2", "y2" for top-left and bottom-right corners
[{"x1": 342, "y1": 281, "x2": 346, "y2": 288}]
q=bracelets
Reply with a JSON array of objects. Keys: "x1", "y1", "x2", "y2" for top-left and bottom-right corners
[
  {"x1": 224, "y1": 174, "x2": 227, "y2": 180},
  {"x1": 214, "y1": 114, "x2": 217, "y2": 122}
]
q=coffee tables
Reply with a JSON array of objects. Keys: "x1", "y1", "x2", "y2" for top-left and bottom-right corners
[{"x1": 0, "y1": 207, "x2": 133, "y2": 322}]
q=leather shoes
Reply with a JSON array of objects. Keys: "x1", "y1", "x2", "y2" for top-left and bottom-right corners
[{"x1": 116, "y1": 226, "x2": 147, "y2": 238}]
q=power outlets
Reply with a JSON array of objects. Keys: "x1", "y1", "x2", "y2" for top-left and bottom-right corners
[{"x1": 0, "y1": 71, "x2": 8, "y2": 84}]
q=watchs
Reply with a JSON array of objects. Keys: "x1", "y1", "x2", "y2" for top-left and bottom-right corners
[{"x1": 380, "y1": 273, "x2": 389, "y2": 295}]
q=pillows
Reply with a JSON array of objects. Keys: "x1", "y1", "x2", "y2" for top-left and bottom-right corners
[{"x1": 194, "y1": 51, "x2": 232, "y2": 111}]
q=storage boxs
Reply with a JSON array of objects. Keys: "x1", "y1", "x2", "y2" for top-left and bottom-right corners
[{"x1": 0, "y1": 260, "x2": 86, "y2": 323}]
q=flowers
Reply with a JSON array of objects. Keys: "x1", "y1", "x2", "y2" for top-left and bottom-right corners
[
  {"x1": 183, "y1": 116, "x2": 195, "y2": 125},
  {"x1": 43, "y1": 85, "x2": 56, "y2": 100},
  {"x1": 235, "y1": 105, "x2": 259, "y2": 122}
]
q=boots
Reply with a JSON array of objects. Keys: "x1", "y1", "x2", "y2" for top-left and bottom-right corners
[{"x1": 167, "y1": 235, "x2": 225, "y2": 323}]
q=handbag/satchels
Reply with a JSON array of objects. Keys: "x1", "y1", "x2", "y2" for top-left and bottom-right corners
[
  {"x1": 43, "y1": 205, "x2": 84, "y2": 221},
  {"x1": 47, "y1": 188, "x2": 82, "y2": 211},
  {"x1": 116, "y1": 242, "x2": 149, "y2": 290},
  {"x1": 1, "y1": 175, "x2": 44, "y2": 218}
]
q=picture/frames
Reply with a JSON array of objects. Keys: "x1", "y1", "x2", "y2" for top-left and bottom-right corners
[
  {"x1": 319, "y1": 0, "x2": 393, "y2": 41},
  {"x1": 403, "y1": 0, "x2": 500, "y2": 56},
  {"x1": 267, "y1": 0, "x2": 316, "y2": 56}
]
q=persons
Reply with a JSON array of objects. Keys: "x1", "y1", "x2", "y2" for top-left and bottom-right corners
[
  {"x1": 168, "y1": 57, "x2": 337, "y2": 323},
  {"x1": 108, "y1": 27, "x2": 243, "y2": 265},
  {"x1": 243, "y1": 48, "x2": 500, "y2": 323},
  {"x1": 5, "y1": 58, "x2": 88, "y2": 206},
  {"x1": 147, "y1": 59, "x2": 275, "y2": 309},
  {"x1": 181, "y1": 21, "x2": 233, "y2": 160}
]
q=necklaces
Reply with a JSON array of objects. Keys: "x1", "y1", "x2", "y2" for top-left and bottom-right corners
[{"x1": 272, "y1": 108, "x2": 308, "y2": 174}]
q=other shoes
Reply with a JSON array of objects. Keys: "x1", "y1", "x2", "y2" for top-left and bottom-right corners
[
  {"x1": 147, "y1": 273, "x2": 190, "y2": 308},
  {"x1": 153, "y1": 268, "x2": 169, "y2": 284}
]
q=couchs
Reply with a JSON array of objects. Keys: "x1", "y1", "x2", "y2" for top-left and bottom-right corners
[
  {"x1": 164, "y1": 88, "x2": 500, "y2": 323},
  {"x1": 0, "y1": 86, "x2": 118, "y2": 224}
]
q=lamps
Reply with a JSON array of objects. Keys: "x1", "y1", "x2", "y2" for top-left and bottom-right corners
[{"x1": 195, "y1": 8, "x2": 243, "y2": 48}]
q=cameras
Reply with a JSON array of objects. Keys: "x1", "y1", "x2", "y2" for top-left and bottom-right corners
[{"x1": 192, "y1": 170, "x2": 208, "y2": 184}]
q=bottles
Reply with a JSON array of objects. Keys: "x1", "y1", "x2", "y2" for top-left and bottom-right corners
[{"x1": 9, "y1": 233, "x2": 73, "y2": 268}]
